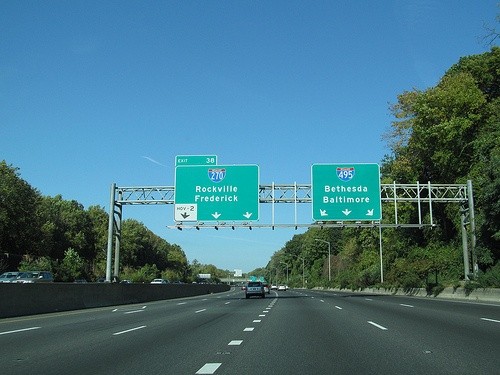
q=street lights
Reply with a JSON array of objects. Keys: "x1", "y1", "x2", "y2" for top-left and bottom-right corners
[
  {"x1": 315, "y1": 239, "x2": 331, "y2": 281},
  {"x1": 265, "y1": 262, "x2": 288, "y2": 286},
  {"x1": 289, "y1": 254, "x2": 304, "y2": 288}
]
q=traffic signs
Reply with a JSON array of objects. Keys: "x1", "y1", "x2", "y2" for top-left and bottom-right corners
[
  {"x1": 311, "y1": 162, "x2": 382, "y2": 221},
  {"x1": 174, "y1": 155, "x2": 260, "y2": 222}
]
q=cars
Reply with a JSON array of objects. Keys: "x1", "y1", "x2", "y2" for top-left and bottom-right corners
[
  {"x1": 241, "y1": 284, "x2": 290, "y2": 294},
  {"x1": 0, "y1": 271, "x2": 222, "y2": 285},
  {"x1": 246, "y1": 281, "x2": 265, "y2": 299}
]
